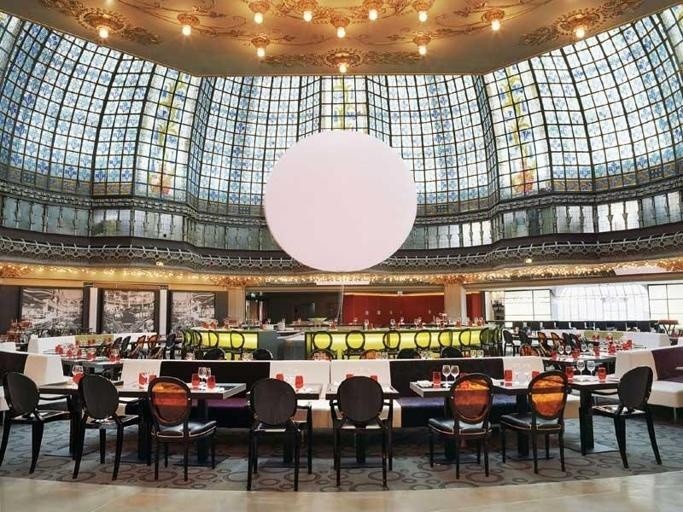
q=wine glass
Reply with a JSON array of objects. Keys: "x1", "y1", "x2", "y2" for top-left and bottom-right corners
[
  {"x1": 267, "y1": 314, "x2": 486, "y2": 358},
  {"x1": 576, "y1": 359, "x2": 585, "y2": 380},
  {"x1": 450, "y1": 365, "x2": 460, "y2": 384},
  {"x1": 53, "y1": 344, "x2": 119, "y2": 364},
  {"x1": 198, "y1": 367, "x2": 206, "y2": 388},
  {"x1": 587, "y1": 360, "x2": 596, "y2": 379},
  {"x1": 442, "y1": 365, "x2": 451, "y2": 387}
]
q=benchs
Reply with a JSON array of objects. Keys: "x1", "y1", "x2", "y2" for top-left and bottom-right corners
[{"x1": 633, "y1": 345, "x2": 683, "y2": 421}]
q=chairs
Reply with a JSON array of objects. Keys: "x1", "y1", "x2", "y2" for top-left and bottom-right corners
[
  {"x1": 72, "y1": 374, "x2": 145, "y2": 481},
  {"x1": 0, "y1": 327, "x2": 662, "y2": 446},
  {"x1": 498, "y1": 369, "x2": 569, "y2": 476},
  {"x1": 246, "y1": 378, "x2": 307, "y2": 491},
  {"x1": 147, "y1": 376, "x2": 217, "y2": 483},
  {"x1": 427, "y1": 373, "x2": 495, "y2": 480},
  {"x1": 578, "y1": 365, "x2": 662, "y2": 469},
  {"x1": 329, "y1": 375, "x2": 395, "y2": 490},
  {"x1": 0, "y1": 372, "x2": 76, "y2": 475}
]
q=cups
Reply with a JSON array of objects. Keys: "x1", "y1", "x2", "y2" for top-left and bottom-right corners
[
  {"x1": 191, "y1": 373, "x2": 200, "y2": 389},
  {"x1": 550, "y1": 340, "x2": 633, "y2": 360},
  {"x1": 276, "y1": 373, "x2": 283, "y2": 381},
  {"x1": 208, "y1": 375, "x2": 216, "y2": 389},
  {"x1": 566, "y1": 366, "x2": 574, "y2": 380},
  {"x1": 597, "y1": 368, "x2": 606, "y2": 382},
  {"x1": 72, "y1": 365, "x2": 84, "y2": 383},
  {"x1": 295, "y1": 375, "x2": 303, "y2": 389},
  {"x1": 433, "y1": 372, "x2": 441, "y2": 386},
  {"x1": 138, "y1": 372, "x2": 157, "y2": 389},
  {"x1": 503, "y1": 368, "x2": 513, "y2": 383},
  {"x1": 204, "y1": 368, "x2": 211, "y2": 381}
]
